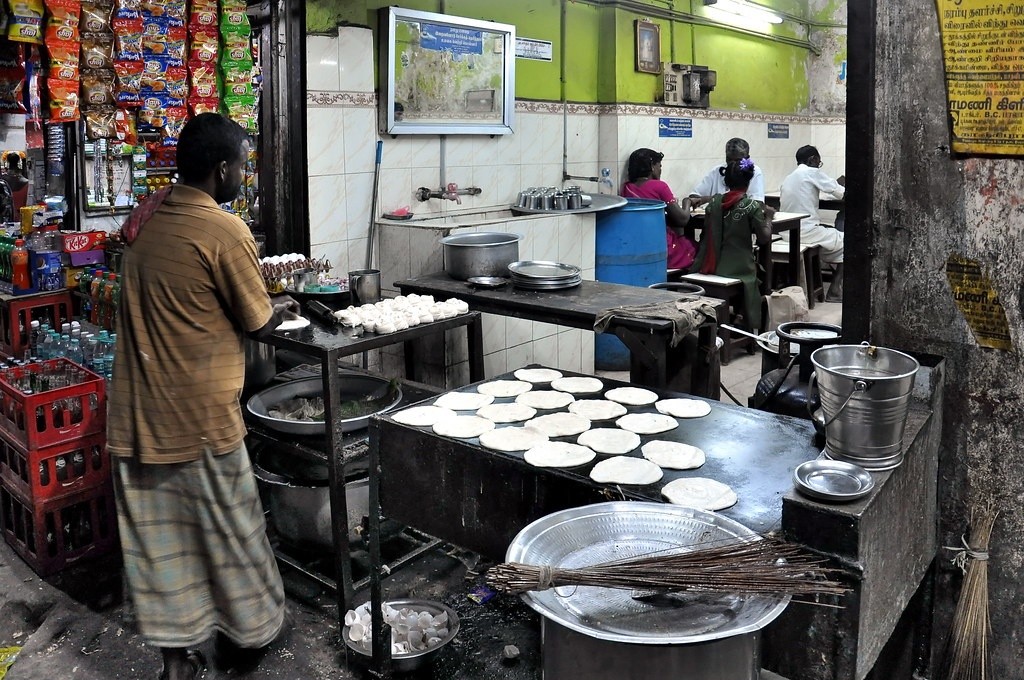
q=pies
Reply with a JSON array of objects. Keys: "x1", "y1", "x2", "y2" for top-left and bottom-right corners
[{"x1": 390, "y1": 368, "x2": 738, "y2": 514}]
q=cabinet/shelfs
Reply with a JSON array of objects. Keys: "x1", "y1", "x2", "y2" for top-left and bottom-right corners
[{"x1": 235, "y1": 307, "x2": 483, "y2": 668}]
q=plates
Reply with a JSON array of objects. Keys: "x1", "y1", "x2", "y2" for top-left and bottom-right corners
[
  {"x1": 468, "y1": 276, "x2": 507, "y2": 286},
  {"x1": 792, "y1": 460, "x2": 875, "y2": 501},
  {"x1": 507, "y1": 260, "x2": 583, "y2": 292}
]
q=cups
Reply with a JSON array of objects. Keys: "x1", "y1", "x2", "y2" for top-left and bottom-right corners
[
  {"x1": 514, "y1": 185, "x2": 592, "y2": 210},
  {"x1": 292, "y1": 267, "x2": 317, "y2": 292},
  {"x1": 348, "y1": 269, "x2": 381, "y2": 306}
]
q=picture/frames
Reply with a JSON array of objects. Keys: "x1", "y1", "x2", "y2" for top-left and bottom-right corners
[{"x1": 633, "y1": 19, "x2": 662, "y2": 74}]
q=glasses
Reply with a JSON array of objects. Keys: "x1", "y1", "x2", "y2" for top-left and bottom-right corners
[{"x1": 814, "y1": 161, "x2": 823, "y2": 167}]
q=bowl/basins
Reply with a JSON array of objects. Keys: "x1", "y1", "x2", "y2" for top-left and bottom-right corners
[
  {"x1": 439, "y1": 232, "x2": 524, "y2": 277},
  {"x1": 342, "y1": 600, "x2": 460, "y2": 671}
]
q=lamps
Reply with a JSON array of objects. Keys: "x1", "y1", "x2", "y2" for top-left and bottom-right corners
[{"x1": 705, "y1": 0, "x2": 783, "y2": 24}]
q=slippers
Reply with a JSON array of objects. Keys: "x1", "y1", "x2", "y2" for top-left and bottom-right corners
[
  {"x1": 187, "y1": 649, "x2": 205, "y2": 679},
  {"x1": 279, "y1": 599, "x2": 296, "y2": 645}
]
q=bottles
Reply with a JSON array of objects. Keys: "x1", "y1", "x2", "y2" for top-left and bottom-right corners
[
  {"x1": 0, "y1": 235, "x2": 121, "y2": 562},
  {"x1": 143, "y1": 141, "x2": 179, "y2": 195}
]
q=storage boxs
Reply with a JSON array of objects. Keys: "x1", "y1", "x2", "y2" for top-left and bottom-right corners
[{"x1": 0, "y1": 227, "x2": 138, "y2": 581}]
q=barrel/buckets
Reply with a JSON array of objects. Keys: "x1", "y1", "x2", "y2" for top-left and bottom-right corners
[
  {"x1": 541, "y1": 614, "x2": 762, "y2": 680},
  {"x1": 630, "y1": 281, "x2": 834, "y2": 401},
  {"x1": 807, "y1": 342, "x2": 919, "y2": 472},
  {"x1": 595, "y1": 197, "x2": 667, "y2": 369},
  {"x1": 249, "y1": 446, "x2": 371, "y2": 545}
]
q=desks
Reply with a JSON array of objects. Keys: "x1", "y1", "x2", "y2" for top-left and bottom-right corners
[
  {"x1": 666, "y1": 209, "x2": 808, "y2": 350},
  {"x1": 394, "y1": 271, "x2": 727, "y2": 393},
  {"x1": 765, "y1": 195, "x2": 846, "y2": 240}
]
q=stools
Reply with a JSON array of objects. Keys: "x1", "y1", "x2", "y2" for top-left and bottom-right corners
[{"x1": 668, "y1": 237, "x2": 824, "y2": 365}]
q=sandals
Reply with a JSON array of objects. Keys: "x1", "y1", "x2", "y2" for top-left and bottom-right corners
[{"x1": 826, "y1": 293, "x2": 842, "y2": 302}]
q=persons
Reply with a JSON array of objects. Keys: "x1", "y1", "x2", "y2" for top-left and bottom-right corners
[
  {"x1": 690, "y1": 138, "x2": 765, "y2": 205},
  {"x1": 777, "y1": 145, "x2": 846, "y2": 303},
  {"x1": 102, "y1": 112, "x2": 300, "y2": 680},
  {"x1": 621, "y1": 148, "x2": 699, "y2": 270},
  {"x1": 692, "y1": 157, "x2": 763, "y2": 327}
]
